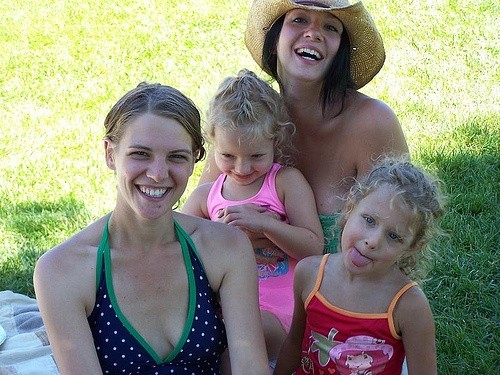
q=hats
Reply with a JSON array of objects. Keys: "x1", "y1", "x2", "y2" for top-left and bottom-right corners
[{"x1": 243, "y1": 0, "x2": 386, "y2": 92}]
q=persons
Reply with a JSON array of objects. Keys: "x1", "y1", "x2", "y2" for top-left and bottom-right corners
[
  {"x1": 181, "y1": 67, "x2": 325, "y2": 364},
  {"x1": 33, "y1": 82, "x2": 269, "y2": 375},
  {"x1": 273, "y1": 151, "x2": 449, "y2": 375},
  {"x1": 199, "y1": 0, "x2": 412, "y2": 260}
]
